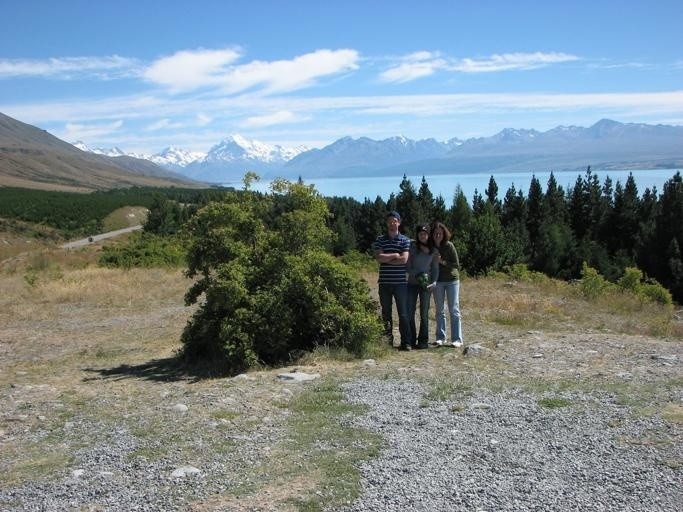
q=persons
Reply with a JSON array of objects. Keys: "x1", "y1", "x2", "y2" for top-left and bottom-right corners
[
  {"x1": 430, "y1": 222, "x2": 464, "y2": 349},
  {"x1": 371, "y1": 212, "x2": 413, "y2": 351},
  {"x1": 406, "y1": 222, "x2": 439, "y2": 351}
]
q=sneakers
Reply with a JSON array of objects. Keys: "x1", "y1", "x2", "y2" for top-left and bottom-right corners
[{"x1": 400, "y1": 340, "x2": 463, "y2": 351}]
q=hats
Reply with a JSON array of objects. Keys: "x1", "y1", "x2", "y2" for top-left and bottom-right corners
[
  {"x1": 386, "y1": 211, "x2": 400, "y2": 219},
  {"x1": 416, "y1": 226, "x2": 429, "y2": 233}
]
